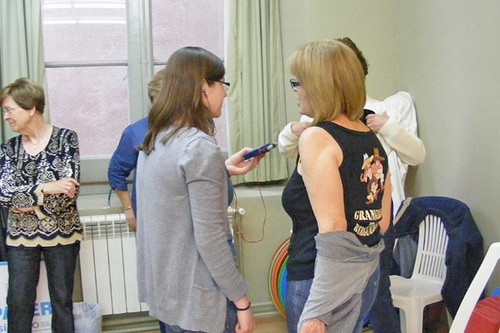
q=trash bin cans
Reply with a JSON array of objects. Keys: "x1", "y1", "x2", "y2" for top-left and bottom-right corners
[{"x1": 72, "y1": 302, "x2": 103, "y2": 333}]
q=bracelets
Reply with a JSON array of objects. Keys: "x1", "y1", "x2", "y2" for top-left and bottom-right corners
[
  {"x1": 122, "y1": 205, "x2": 134, "y2": 212},
  {"x1": 236, "y1": 301, "x2": 251, "y2": 312}
]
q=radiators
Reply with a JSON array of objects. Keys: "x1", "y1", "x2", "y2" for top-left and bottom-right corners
[{"x1": 79, "y1": 206, "x2": 245, "y2": 316}]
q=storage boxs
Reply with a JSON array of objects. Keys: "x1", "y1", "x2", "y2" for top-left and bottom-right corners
[{"x1": 0, "y1": 258, "x2": 53, "y2": 333}]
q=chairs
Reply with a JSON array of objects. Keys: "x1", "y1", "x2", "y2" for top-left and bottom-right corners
[{"x1": 391, "y1": 196, "x2": 500, "y2": 333}]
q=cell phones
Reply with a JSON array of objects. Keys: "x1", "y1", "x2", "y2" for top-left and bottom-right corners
[{"x1": 245, "y1": 142, "x2": 276, "y2": 160}]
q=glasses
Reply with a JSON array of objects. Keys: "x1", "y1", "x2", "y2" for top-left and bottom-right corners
[
  {"x1": 206, "y1": 78, "x2": 231, "y2": 91},
  {"x1": 289, "y1": 79, "x2": 302, "y2": 88}
]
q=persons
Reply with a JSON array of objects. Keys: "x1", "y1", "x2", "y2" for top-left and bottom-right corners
[
  {"x1": 0, "y1": 77, "x2": 83, "y2": 333},
  {"x1": 279, "y1": 37, "x2": 425, "y2": 333},
  {"x1": 107, "y1": 66, "x2": 243, "y2": 333},
  {"x1": 282, "y1": 38, "x2": 394, "y2": 333},
  {"x1": 131, "y1": 45, "x2": 259, "y2": 333}
]
